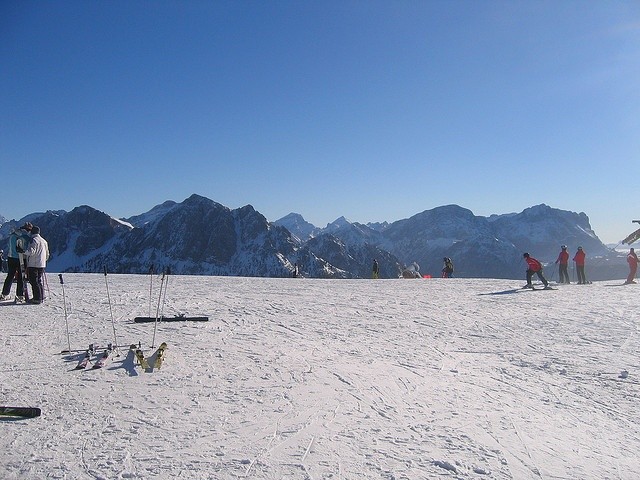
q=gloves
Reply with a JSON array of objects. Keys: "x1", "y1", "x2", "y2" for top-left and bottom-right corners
[{"x1": 16, "y1": 246, "x2": 25, "y2": 253}]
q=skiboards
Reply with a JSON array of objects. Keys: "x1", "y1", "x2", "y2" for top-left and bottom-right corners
[
  {"x1": 0, "y1": 406, "x2": 42, "y2": 418},
  {"x1": 569, "y1": 279, "x2": 593, "y2": 286},
  {"x1": 135, "y1": 314, "x2": 209, "y2": 322},
  {"x1": 521, "y1": 286, "x2": 559, "y2": 290},
  {"x1": 130, "y1": 342, "x2": 167, "y2": 369},
  {"x1": 76, "y1": 344, "x2": 117, "y2": 369},
  {"x1": 17, "y1": 238, "x2": 29, "y2": 302}
]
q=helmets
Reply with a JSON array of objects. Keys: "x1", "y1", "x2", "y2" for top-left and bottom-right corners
[
  {"x1": 523, "y1": 253, "x2": 529, "y2": 257},
  {"x1": 561, "y1": 245, "x2": 567, "y2": 250},
  {"x1": 577, "y1": 247, "x2": 582, "y2": 250}
]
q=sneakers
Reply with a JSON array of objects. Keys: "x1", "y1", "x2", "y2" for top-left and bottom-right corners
[
  {"x1": 15, "y1": 296, "x2": 26, "y2": 304},
  {"x1": 543, "y1": 284, "x2": 551, "y2": 289},
  {"x1": 26, "y1": 299, "x2": 44, "y2": 304},
  {"x1": 1, "y1": 294, "x2": 10, "y2": 301},
  {"x1": 524, "y1": 284, "x2": 532, "y2": 289}
]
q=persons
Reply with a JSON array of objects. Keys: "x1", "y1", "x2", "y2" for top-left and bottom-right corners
[
  {"x1": 573, "y1": 246, "x2": 585, "y2": 284},
  {"x1": 17, "y1": 227, "x2": 50, "y2": 304},
  {"x1": 293, "y1": 263, "x2": 299, "y2": 277},
  {"x1": 0, "y1": 222, "x2": 32, "y2": 303},
  {"x1": 524, "y1": 253, "x2": 548, "y2": 288},
  {"x1": 0, "y1": 250, "x2": 8, "y2": 271},
  {"x1": 373, "y1": 259, "x2": 379, "y2": 279},
  {"x1": 626, "y1": 248, "x2": 640, "y2": 283},
  {"x1": 442, "y1": 257, "x2": 454, "y2": 278},
  {"x1": 555, "y1": 245, "x2": 570, "y2": 284}
]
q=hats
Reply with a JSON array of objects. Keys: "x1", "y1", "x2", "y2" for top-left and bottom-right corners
[
  {"x1": 31, "y1": 226, "x2": 40, "y2": 234},
  {"x1": 25, "y1": 222, "x2": 33, "y2": 230}
]
